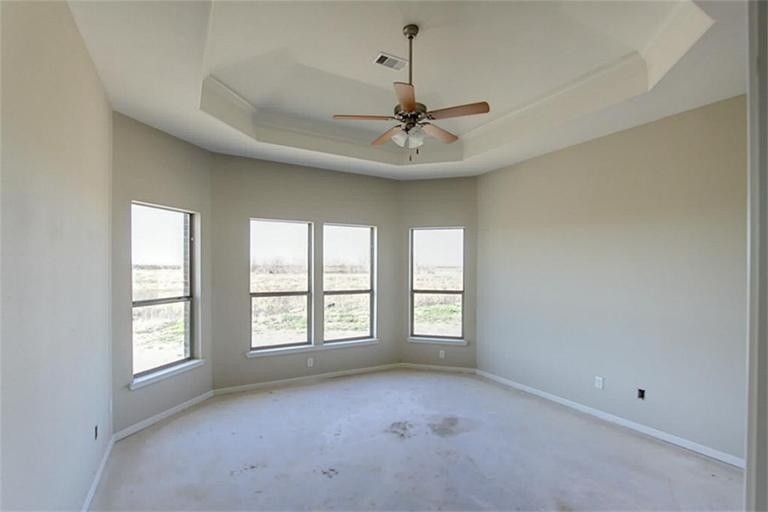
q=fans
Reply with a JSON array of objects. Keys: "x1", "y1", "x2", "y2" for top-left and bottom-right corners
[{"x1": 333, "y1": 24, "x2": 490, "y2": 148}]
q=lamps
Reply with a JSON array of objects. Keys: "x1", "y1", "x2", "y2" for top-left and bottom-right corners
[{"x1": 391, "y1": 118, "x2": 424, "y2": 161}]
